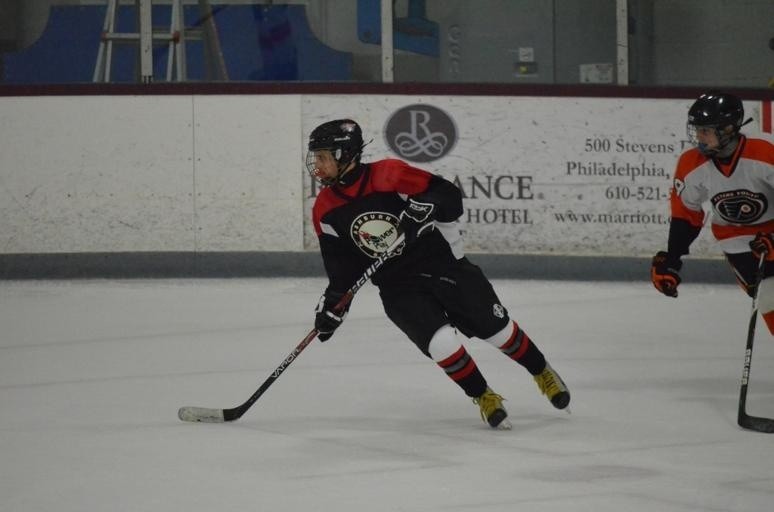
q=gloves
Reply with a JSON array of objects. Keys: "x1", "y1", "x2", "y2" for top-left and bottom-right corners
[
  {"x1": 396, "y1": 193, "x2": 440, "y2": 257},
  {"x1": 748, "y1": 231, "x2": 774, "y2": 263},
  {"x1": 312, "y1": 288, "x2": 352, "y2": 342},
  {"x1": 651, "y1": 250, "x2": 683, "y2": 299}
]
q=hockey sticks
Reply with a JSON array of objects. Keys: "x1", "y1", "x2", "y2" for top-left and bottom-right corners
[
  {"x1": 738, "y1": 253, "x2": 774, "y2": 433},
  {"x1": 178, "y1": 232, "x2": 406, "y2": 423}
]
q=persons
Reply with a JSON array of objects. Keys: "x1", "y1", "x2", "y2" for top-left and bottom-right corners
[
  {"x1": 650, "y1": 90, "x2": 773, "y2": 340},
  {"x1": 307, "y1": 116, "x2": 571, "y2": 430}
]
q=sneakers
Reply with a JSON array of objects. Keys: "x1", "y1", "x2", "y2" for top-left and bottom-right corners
[
  {"x1": 474, "y1": 385, "x2": 508, "y2": 428},
  {"x1": 533, "y1": 359, "x2": 570, "y2": 410}
]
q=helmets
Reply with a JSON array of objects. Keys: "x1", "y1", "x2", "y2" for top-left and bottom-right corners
[
  {"x1": 305, "y1": 118, "x2": 364, "y2": 187},
  {"x1": 685, "y1": 90, "x2": 744, "y2": 161}
]
